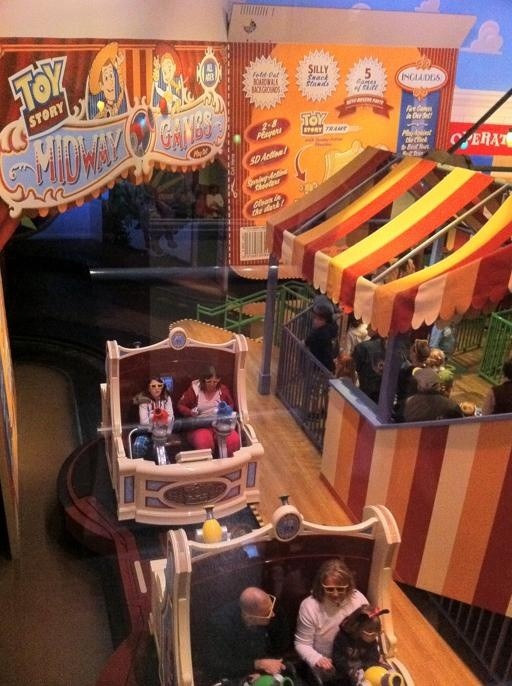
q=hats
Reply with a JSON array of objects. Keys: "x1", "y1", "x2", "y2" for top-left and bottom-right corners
[
  {"x1": 412, "y1": 366, "x2": 440, "y2": 388},
  {"x1": 311, "y1": 303, "x2": 332, "y2": 321}
]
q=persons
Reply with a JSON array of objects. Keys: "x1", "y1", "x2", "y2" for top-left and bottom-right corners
[
  {"x1": 293, "y1": 558, "x2": 370, "y2": 685},
  {"x1": 154, "y1": 185, "x2": 225, "y2": 219},
  {"x1": 132, "y1": 376, "x2": 175, "y2": 462},
  {"x1": 176, "y1": 366, "x2": 240, "y2": 459},
  {"x1": 333, "y1": 605, "x2": 394, "y2": 686},
  {"x1": 295, "y1": 248, "x2": 512, "y2": 433},
  {"x1": 201, "y1": 586, "x2": 296, "y2": 686}
]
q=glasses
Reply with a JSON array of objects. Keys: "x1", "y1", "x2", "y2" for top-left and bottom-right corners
[
  {"x1": 322, "y1": 584, "x2": 348, "y2": 594},
  {"x1": 149, "y1": 384, "x2": 164, "y2": 388},
  {"x1": 362, "y1": 630, "x2": 384, "y2": 636},
  {"x1": 244, "y1": 594, "x2": 277, "y2": 620},
  {"x1": 205, "y1": 380, "x2": 219, "y2": 384}
]
